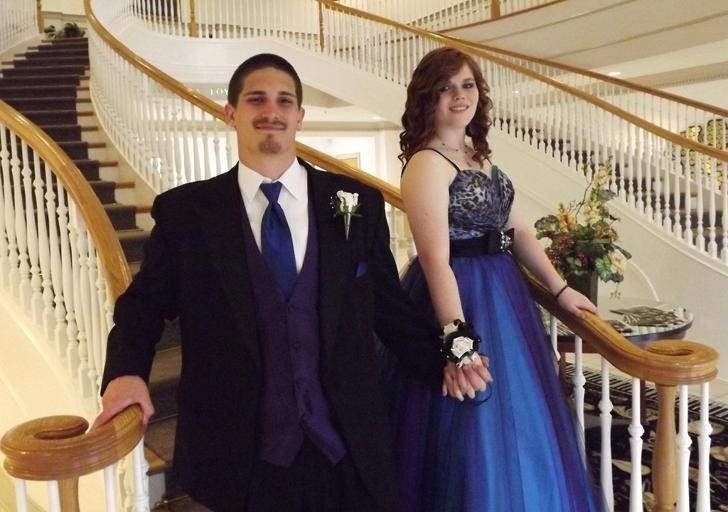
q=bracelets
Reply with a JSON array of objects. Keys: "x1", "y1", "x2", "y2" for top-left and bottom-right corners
[
  {"x1": 439, "y1": 320, "x2": 480, "y2": 366},
  {"x1": 555, "y1": 284, "x2": 569, "y2": 300}
]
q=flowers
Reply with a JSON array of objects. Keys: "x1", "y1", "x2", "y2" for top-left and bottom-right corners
[
  {"x1": 329, "y1": 190, "x2": 366, "y2": 243},
  {"x1": 534, "y1": 154, "x2": 634, "y2": 300},
  {"x1": 435, "y1": 318, "x2": 482, "y2": 369}
]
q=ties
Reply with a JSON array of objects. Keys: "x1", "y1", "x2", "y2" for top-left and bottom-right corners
[{"x1": 258, "y1": 181, "x2": 299, "y2": 299}]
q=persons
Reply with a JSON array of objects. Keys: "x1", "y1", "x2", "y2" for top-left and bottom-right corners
[
  {"x1": 89, "y1": 53, "x2": 493, "y2": 510},
  {"x1": 395, "y1": 48, "x2": 602, "y2": 512}
]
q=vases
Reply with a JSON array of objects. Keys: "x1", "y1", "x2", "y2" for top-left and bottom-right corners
[{"x1": 567, "y1": 273, "x2": 597, "y2": 309}]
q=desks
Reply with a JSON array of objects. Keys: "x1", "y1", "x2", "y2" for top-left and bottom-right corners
[{"x1": 540, "y1": 300, "x2": 695, "y2": 442}]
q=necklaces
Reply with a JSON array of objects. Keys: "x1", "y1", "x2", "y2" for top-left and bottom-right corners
[{"x1": 438, "y1": 133, "x2": 474, "y2": 169}]
